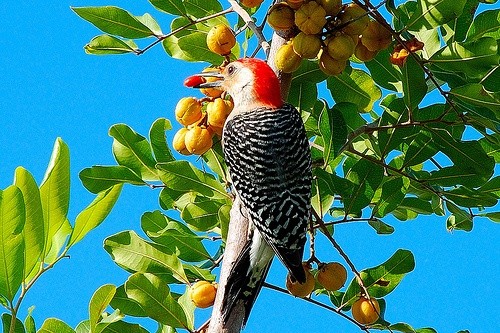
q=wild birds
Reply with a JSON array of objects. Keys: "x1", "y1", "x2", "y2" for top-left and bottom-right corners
[{"x1": 193, "y1": 59, "x2": 312, "y2": 331}]
